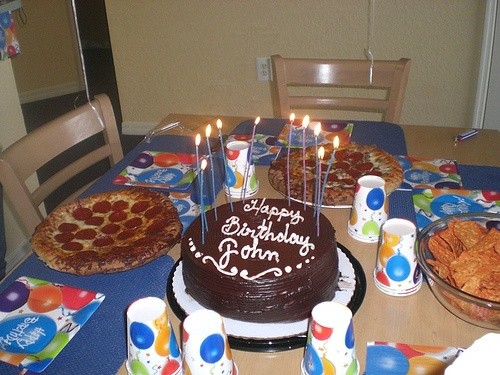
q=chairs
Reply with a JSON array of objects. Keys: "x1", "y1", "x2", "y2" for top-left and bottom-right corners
[
  {"x1": 0, "y1": 92, "x2": 126, "y2": 239},
  {"x1": 270, "y1": 54, "x2": 412, "y2": 126}
]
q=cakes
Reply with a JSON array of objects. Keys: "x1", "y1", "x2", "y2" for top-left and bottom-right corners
[{"x1": 180, "y1": 197, "x2": 340, "y2": 324}]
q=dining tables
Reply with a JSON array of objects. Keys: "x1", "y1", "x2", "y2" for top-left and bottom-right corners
[{"x1": 0, "y1": 110, "x2": 500, "y2": 375}]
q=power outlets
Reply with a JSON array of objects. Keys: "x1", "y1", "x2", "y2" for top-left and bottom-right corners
[{"x1": 254, "y1": 57, "x2": 272, "y2": 82}]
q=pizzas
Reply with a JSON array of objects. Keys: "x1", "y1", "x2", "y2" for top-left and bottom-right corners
[
  {"x1": 268, "y1": 142, "x2": 403, "y2": 206},
  {"x1": 30, "y1": 189, "x2": 183, "y2": 275}
]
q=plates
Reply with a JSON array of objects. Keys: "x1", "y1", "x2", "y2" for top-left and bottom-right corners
[
  {"x1": 273, "y1": 120, "x2": 354, "y2": 148},
  {"x1": 112, "y1": 151, "x2": 211, "y2": 190},
  {"x1": 207, "y1": 135, "x2": 283, "y2": 164},
  {"x1": 390, "y1": 155, "x2": 500, "y2": 231},
  {"x1": 364, "y1": 340, "x2": 467, "y2": 375},
  {"x1": 0, "y1": 276, "x2": 107, "y2": 373}
]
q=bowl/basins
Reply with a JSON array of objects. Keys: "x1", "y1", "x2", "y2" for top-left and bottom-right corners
[{"x1": 415, "y1": 212, "x2": 500, "y2": 329}]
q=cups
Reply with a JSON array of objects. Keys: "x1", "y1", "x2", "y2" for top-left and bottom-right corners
[
  {"x1": 374, "y1": 218, "x2": 423, "y2": 296},
  {"x1": 222, "y1": 141, "x2": 259, "y2": 198},
  {"x1": 183, "y1": 309, "x2": 238, "y2": 375},
  {"x1": 300, "y1": 301, "x2": 361, "y2": 375},
  {"x1": 347, "y1": 175, "x2": 389, "y2": 243},
  {"x1": 125, "y1": 297, "x2": 182, "y2": 375}
]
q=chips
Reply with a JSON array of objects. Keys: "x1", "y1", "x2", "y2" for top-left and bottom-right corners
[{"x1": 426, "y1": 217, "x2": 500, "y2": 322}]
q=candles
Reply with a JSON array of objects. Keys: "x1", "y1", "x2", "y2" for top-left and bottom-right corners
[{"x1": 194, "y1": 113, "x2": 340, "y2": 246}]
